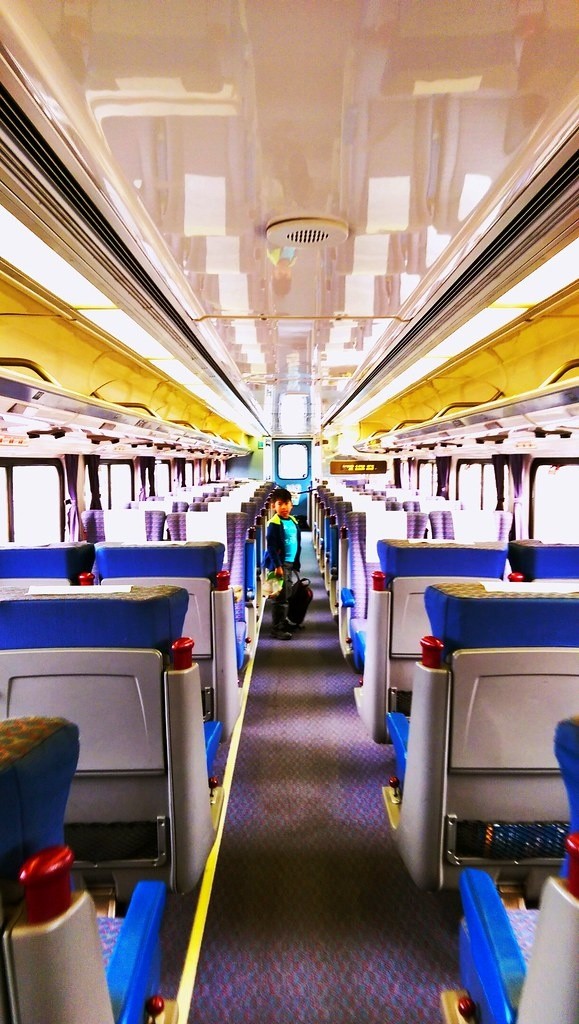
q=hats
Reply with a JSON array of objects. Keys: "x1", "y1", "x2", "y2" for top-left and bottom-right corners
[{"x1": 262, "y1": 572, "x2": 284, "y2": 600}]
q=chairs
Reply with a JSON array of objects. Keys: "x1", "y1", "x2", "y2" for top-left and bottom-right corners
[{"x1": 0, "y1": 478, "x2": 579, "y2": 1024}]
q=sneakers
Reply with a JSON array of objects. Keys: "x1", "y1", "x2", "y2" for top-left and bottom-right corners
[
  {"x1": 270, "y1": 630, "x2": 292, "y2": 640},
  {"x1": 285, "y1": 619, "x2": 305, "y2": 632}
]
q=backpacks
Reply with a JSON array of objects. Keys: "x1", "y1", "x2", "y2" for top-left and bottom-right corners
[{"x1": 286, "y1": 570, "x2": 313, "y2": 624}]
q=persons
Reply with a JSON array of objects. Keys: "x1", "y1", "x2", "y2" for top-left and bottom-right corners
[{"x1": 262, "y1": 489, "x2": 302, "y2": 640}]
